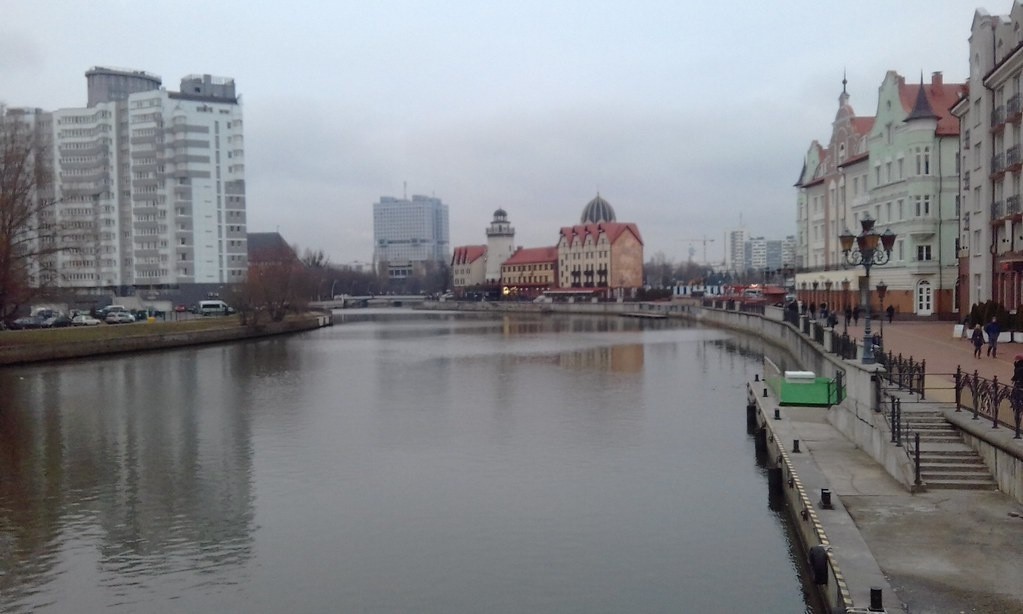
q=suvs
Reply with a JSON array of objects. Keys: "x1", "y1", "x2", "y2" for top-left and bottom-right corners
[{"x1": 97, "y1": 304, "x2": 135, "y2": 324}]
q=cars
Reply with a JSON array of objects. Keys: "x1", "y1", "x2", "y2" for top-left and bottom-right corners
[{"x1": 14, "y1": 307, "x2": 102, "y2": 329}]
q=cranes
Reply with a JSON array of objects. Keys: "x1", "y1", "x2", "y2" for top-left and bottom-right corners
[{"x1": 688, "y1": 235, "x2": 716, "y2": 261}]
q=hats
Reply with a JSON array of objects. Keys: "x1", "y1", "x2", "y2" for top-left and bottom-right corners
[{"x1": 1013, "y1": 354, "x2": 1023, "y2": 360}]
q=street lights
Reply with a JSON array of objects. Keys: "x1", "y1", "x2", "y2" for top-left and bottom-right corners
[
  {"x1": 876, "y1": 278, "x2": 887, "y2": 338},
  {"x1": 776, "y1": 261, "x2": 795, "y2": 288},
  {"x1": 838, "y1": 213, "x2": 897, "y2": 365},
  {"x1": 812, "y1": 280, "x2": 819, "y2": 306},
  {"x1": 840, "y1": 277, "x2": 850, "y2": 337},
  {"x1": 825, "y1": 279, "x2": 832, "y2": 313},
  {"x1": 801, "y1": 282, "x2": 807, "y2": 304}
]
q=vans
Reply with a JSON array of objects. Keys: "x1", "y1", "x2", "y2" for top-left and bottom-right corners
[{"x1": 199, "y1": 300, "x2": 233, "y2": 315}]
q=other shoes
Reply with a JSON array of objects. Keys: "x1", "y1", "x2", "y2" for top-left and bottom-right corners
[
  {"x1": 974, "y1": 353, "x2": 977, "y2": 357},
  {"x1": 992, "y1": 353, "x2": 996, "y2": 358},
  {"x1": 978, "y1": 355, "x2": 980, "y2": 359},
  {"x1": 987, "y1": 351, "x2": 990, "y2": 356}
]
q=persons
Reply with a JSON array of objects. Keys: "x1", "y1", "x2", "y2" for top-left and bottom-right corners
[
  {"x1": 802, "y1": 300, "x2": 861, "y2": 328},
  {"x1": 971, "y1": 324, "x2": 986, "y2": 360},
  {"x1": 887, "y1": 304, "x2": 894, "y2": 323},
  {"x1": 985, "y1": 314, "x2": 1000, "y2": 359},
  {"x1": 1010, "y1": 354, "x2": 1023, "y2": 400}
]
